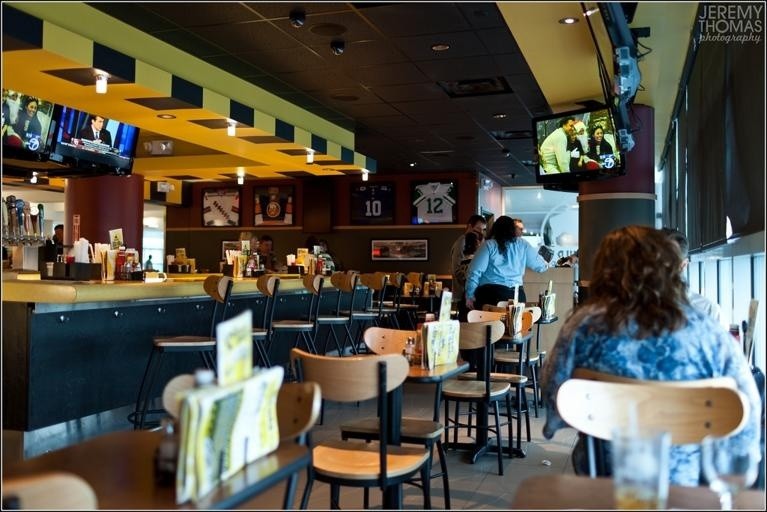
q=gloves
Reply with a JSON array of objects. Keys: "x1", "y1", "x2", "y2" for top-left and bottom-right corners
[{"x1": 571, "y1": 147, "x2": 580, "y2": 158}]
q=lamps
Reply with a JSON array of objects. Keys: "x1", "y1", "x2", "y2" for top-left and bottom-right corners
[
  {"x1": 95, "y1": 73, "x2": 108, "y2": 94},
  {"x1": 227, "y1": 121, "x2": 236, "y2": 136},
  {"x1": 306, "y1": 150, "x2": 314, "y2": 165},
  {"x1": 238, "y1": 167, "x2": 245, "y2": 185},
  {"x1": 361, "y1": 168, "x2": 369, "y2": 182}
]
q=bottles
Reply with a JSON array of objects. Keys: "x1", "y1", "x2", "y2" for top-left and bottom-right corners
[
  {"x1": 152, "y1": 420, "x2": 179, "y2": 487},
  {"x1": 425, "y1": 313, "x2": 435, "y2": 322},
  {"x1": 245, "y1": 253, "x2": 259, "y2": 277},
  {"x1": 500, "y1": 298, "x2": 515, "y2": 336},
  {"x1": 403, "y1": 337, "x2": 414, "y2": 363},
  {"x1": 115, "y1": 246, "x2": 142, "y2": 280},
  {"x1": 415, "y1": 324, "x2": 421, "y2": 365},
  {"x1": 194, "y1": 368, "x2": 217, "y2": 388},
  {"x1": 316, "y1": 255, "x2": 328, "y2": 275},
  {"x1": 536, "y1": 290, "x2": 543, "y2": 320}
]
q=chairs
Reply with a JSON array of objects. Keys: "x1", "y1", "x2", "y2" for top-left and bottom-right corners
[
  {"x1": 558, "y1": 378, "x2": 751, "y2": 485},
  {"x1": 571, "y1": 366, "x2": 740, "y2": 391}
]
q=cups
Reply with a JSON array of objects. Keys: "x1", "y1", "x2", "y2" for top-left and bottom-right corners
[{"x1": 613, "y1": 399, "x2": 670, "y2": 509}]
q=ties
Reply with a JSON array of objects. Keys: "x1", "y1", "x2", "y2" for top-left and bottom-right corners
[{"x1": 96, "y1": 132, "x2": 98, "y2": 138}]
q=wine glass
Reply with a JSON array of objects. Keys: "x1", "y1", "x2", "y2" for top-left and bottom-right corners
[{"x1": 703, "y1": 434, "x2": 758, "y2": 509}]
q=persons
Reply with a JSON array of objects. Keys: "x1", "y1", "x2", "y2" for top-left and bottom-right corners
[
  {"x1": 77, "y1": 115, "x2": 112, "y2": 146},
  {"x1": 54, "y1": 224, "x2": 64, "y2": 258},
  {"x1": 3, "y1": 88, "x2": 41, "y2": 145},
  {"x1": 258, "y1": 234, "x2": 288, "y2": 274},
  {"x1": 464, "y1": 216, "x2": 548, "y2": 312},
  {"x1": 539, "y1": 117, "x2": 614, "y2": 172},
  {"x1": 456, "y1": 229, "x2": 484, "y2": 300},
  {"x1": 515, "y1": 219, "x2": 524, "y2": 239},
  {"x1": 538, "y1": 224, "x2": 763, "y2": 488},
  {"x1": 659, "y1": 228, "x2": 729, "y2": 331},
  {"x1": 450, "y1": 217, "x2": 488, "y2": 322}
]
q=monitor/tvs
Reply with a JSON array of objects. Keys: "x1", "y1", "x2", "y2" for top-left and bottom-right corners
[
  {"x1": 54, "y1": 102, "x2": 139, "y2": 176},
  {"x1": 532, "y1": 104, "x2": 627, "y2": 183},
  {"x1": 2, "y1": 89, "x2": 54, "y2": 163}
]
